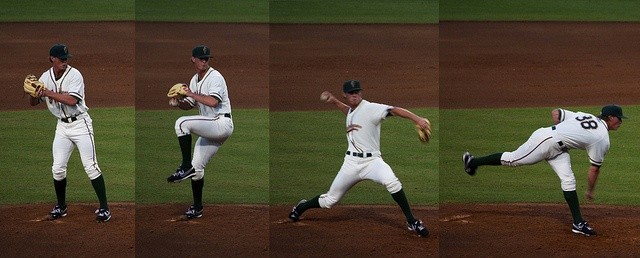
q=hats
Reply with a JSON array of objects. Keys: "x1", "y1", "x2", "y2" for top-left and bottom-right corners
[
  {"x1": 192, "y1": 45, "x2": 212, "y2": 59},
  {"x1": 343, "y1": 80, "x2": 363, "y2": 93},
  {"x1": 600, "y1": 106, "x2": 629, "y2": 119},
  {"x1": 50, "y1": 44, "x2": 73, "y2": 58}
]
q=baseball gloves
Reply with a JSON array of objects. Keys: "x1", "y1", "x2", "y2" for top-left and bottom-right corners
[
  {"x1": 23, "y1": 74, "x2": 45, "y2": 98},
  {"x1": 416, "y1": 118, "x2": 431, "y2": 142},
  {"x1": 167, "y1": 82, "x2": 189, "y2": 98}
]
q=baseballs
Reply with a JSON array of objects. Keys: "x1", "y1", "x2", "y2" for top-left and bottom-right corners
[
  {"x1": 320, "y1": 95, "x2": 328, "y2": 101},
  {"x1": 169, "y1": 99, "x2": 178, "y2": 107}
]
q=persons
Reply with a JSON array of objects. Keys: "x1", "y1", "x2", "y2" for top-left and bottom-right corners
[
  {"x1": 166, "y1": 44, "x2": 233, "y2": 222},
  {"x1": 287, "y1": 80, "x2": 431, "y2": 240},
  {"x1": 21, "y1": 44, "x2": 112, "y2": 222},
  {"x1": 463, "y1": 104, "x2": 624, "y2": 237}
]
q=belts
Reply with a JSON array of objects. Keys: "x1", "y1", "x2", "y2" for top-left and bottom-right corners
[
  {"x1": 552, "y1": 126, "x2": 569, "y2": 153},
  {"x1": 224, "y1": 113, "x2": 232, "y2": 117},
  {"x1": 346, "y1": 151, "x2": 372, "y2": 157},
  {"x1": 61, "y1": 117, "x2": 76, "y2": 123}
]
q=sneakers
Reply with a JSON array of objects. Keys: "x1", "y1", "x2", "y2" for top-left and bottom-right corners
[
  {"x1": 167, "y1": 166, "x2": 196, "y2": 183},
  {"x1": 572, "y1": 221, "x2": 596, "y2": 236},
  {"x1": 463, "y1": 152, "x2": 475, "y2": 176},
  {"x1": 47, "y1": 206, "x2": 68, "y2": 221},
  {"x1": 94, "y1": 209, "x2": 111, "y2": 223},
  {"x1": 407, "y1": 219, "x2": 429, "y2": 238},
  {"x1": 288, "y1": 199, "x2": 308, "y2": 222},
  {"x1": 184, "y1": 206, "x2": 204, "y2": 218}
]
